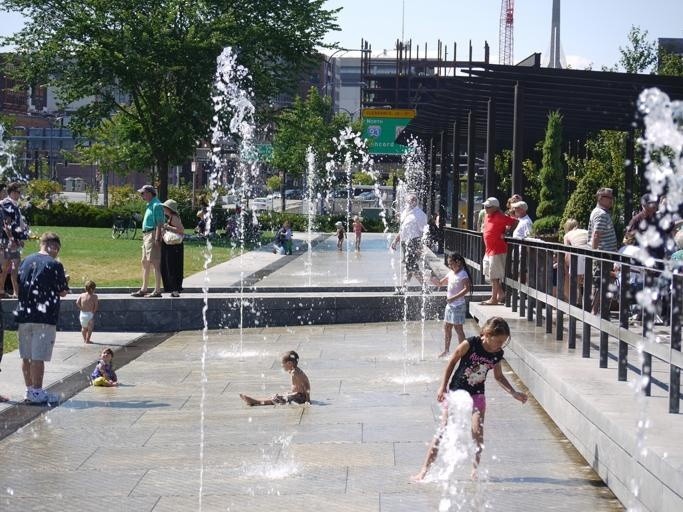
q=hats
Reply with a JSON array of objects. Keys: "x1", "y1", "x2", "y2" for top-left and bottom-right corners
[
  {"x1": 158, "y1": 199, "x2": 177, "y2": 213},
  {"x1": 511, "y1": 201, "x2": 529, "y2": 211},
  {"x1": 481, "y1": 196, "x2": 499, "y2": 207},
  {"x1": 137, "y1": 185, "x2": 155, "y2": 193}
]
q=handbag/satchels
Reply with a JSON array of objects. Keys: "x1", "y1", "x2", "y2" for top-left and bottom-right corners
[{"x1": 161, "y1": 227, "x2": 186, "y2": 245}]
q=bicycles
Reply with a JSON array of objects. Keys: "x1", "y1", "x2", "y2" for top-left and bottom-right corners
[{"x1": 110, "y1": 208, "x2": 142, "y2": 240}]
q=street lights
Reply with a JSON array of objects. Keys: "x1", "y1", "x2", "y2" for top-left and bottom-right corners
[{"x1": 319, "y1": 43, "x2": 391, "y2": 123}]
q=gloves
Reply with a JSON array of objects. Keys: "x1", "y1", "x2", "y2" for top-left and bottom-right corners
[{"x1": 164, "y1": 223, "x2": 176, "y2": 233}]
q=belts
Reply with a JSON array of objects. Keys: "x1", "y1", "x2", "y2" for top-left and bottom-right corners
[{"x1": 143, "y1": 230, "x2": 151, "y2": 233}]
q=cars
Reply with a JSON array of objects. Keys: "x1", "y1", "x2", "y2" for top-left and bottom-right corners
[
  {"x1": 252, "y1": 198, "x2": 269, "y2": 210},
  {"x1": 237, "y1": 185, "x2": 395, "y2": 199}
]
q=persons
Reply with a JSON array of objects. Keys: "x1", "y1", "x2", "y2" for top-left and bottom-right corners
[
  {"x1": 16, "y1": 231, "x2": 68, "y2": 405},
  {"x1": 132, "y1": 185, "x2": 162, "y2": 298},
  {"x1": 390, "y1": 194, "x2": 432, "y2": 296},
  {"x1": 89, "y1": 348, "x2": 118, "y2": 386},
  {"x1": 511, "y1": 201, "x2": 530, "y2": 238},
  {"x1": 334, "y1": 222, "x2": 347, "y2": 251},
  {"x1": 611, "y1": 234, "x2": 643, "y2": 325},
  {"x1": 76, "y1": 280, "x2": 97, "y2": 343},
  {"x1": 587, "y1": 188, "x2": 618, "y2": 316},
  {"x1": 160, "y1": 199, "x2": 182, "y2": 297},
  {"x1": 351, "y1": 216, "x2": 367, "y2": 252},
  {"x1": 413, "y1": 316, "x2": 528, "y2": 480},
  {"x1": 238, "y1": 350, "x2": 310, "y2": 406},
  {"x1": 196, "y1": 191, "x2": 262, "y2": 243},
  {"x1": 272, "y1": 220, "x2": 293, "y2": 255},
  {"x1": 429, "y1": 251, "x2": 473, "y2": 358},
  {"x1": 503, "y1": 195, "x2": 522, "y2": 236},
  {"x1": 0, "y1": 183, "x2": 34, "y2": 296},
  {"x1": 563, "y1": 219, "x2": 589, "y2": 276},
  {"x1": 622, "y1": 194, "x2": 654, "y2": 246},
  {"x1": 480, "y1": 198, "x2": 519, "y2": 305}
]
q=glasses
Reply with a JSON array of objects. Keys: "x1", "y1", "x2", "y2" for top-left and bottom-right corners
[
  {"x1": 602, "y1": 196, "x2": 613, "y2": 200},
  {"x1": 15, "y1": 191, "x2": 20, "y2": 194}
]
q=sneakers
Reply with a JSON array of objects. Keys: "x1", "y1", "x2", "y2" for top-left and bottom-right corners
[
  {"x1": 131, "y1": 289, "x2": 148, "y2": 297},
  {"x1": 171, "y1": 291, "x2": 180, "y2": 297},
  {"x1": 482, "y1": 297, "x2": 498, "y2": 304},
  {"x1": 0, "y1": 394, "x2": 9, "y2": 402},
  {"x1": 148, "y1": 288, "x2": 161, "y2": 297},
  {"x1": 30, "y1": 389, "x2": 59, "y2": 405},
  {"x1": 0, "y1": 292, "x2": 12, "y2": 298},
  {"x1": 24, "y1": 389, "x2": 33, "y2": 404}
]
